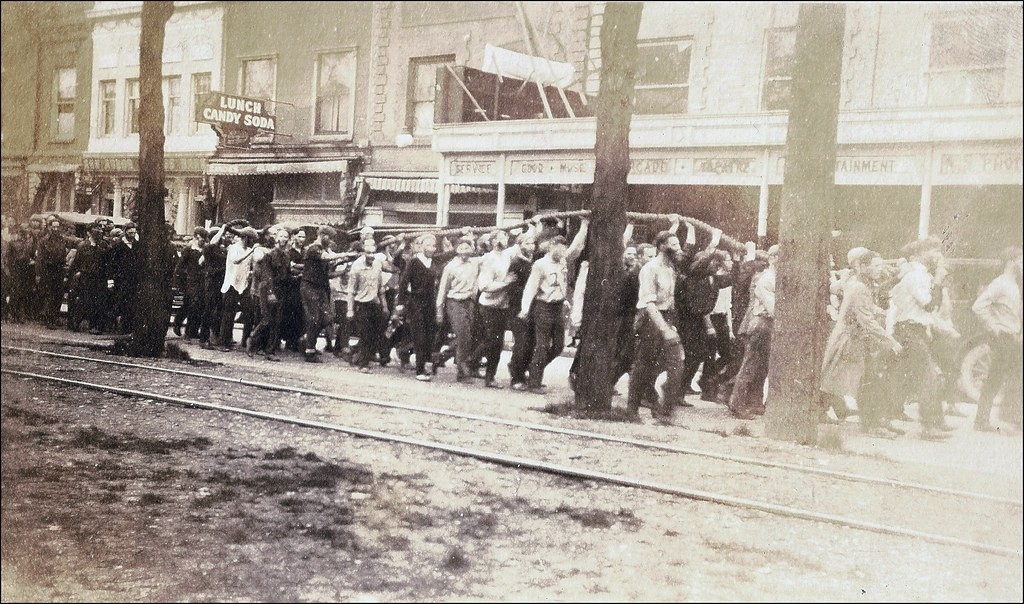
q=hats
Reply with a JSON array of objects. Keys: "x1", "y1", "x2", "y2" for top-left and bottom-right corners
[
  {"x1": 95, "y1": 216, "x2": 106, "y2": 223},
  {"x1": 317, "y1": 224, "x2": 338, "y2": 238}
]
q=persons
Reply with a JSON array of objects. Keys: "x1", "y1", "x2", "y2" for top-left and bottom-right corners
[
  {"x1": 431, "y1": 240, "x2": 484, "y2": 383},
  {"x1": 820, "y1": 247, "x2": 903, "y2": 439},
  {"x1": 300, "y1": 225, "x2": 353, "y2": 362},
  {"x1": 347, "y1": 246, "x2": 391, "y2": 373},
  {"x1": 246, "y1": 231, "x2": 302, "y2": 361},
  {"x1": 626, "y1": 215, "x2": 684, "y2": 427},
  {"x1": 970, "y1": 247, "x2": 1024, "y2": 432},
  {"x1": 457, "y1": 229, "x2": 520, "y2": 389},
  {"x1": 518, "y1": 217, "x2": 590, "y2": 394},
  {"x1": 396, "y1": 235, "x2": 456, "y2": 380},
  {"x1": 1, "y1": 213, "x2": 966, "y2": 440}
]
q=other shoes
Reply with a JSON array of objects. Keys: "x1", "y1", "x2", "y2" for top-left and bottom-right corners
[
  {"x1": 305, "y1": 354, "x2": 324, "y2": 364},
  {"x1": 487, "y1": 381, "x2": 504, "y2": 389},
  {"x1": 458, "y1": 377, "x2": 474, "y2": 385},
  {"x1": 514, "y1": 382, "x2": 528, "y2": 391},
  {"x1": 530, "y1": 386, "x2": 547, "y2": 394},
  {"x1": 246, "y1": 337, "x2": 255, "y2": 357},
  {"x1": 264, "y1": 352, "x2": 281, "y2": 362},
  {"x1": 416, "y1": 374, "x2": 431, "y2": 382}
]
